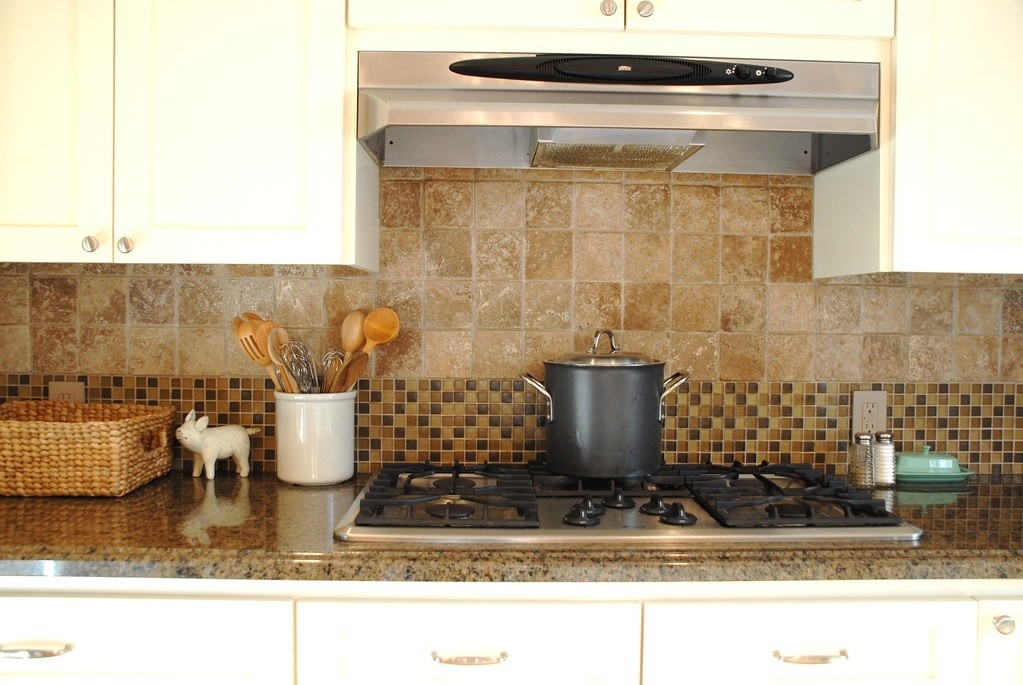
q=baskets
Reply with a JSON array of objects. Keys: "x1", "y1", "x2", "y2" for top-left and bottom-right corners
[{"x1": 0, "y1": 399, "x2": 176, "y2": 497}]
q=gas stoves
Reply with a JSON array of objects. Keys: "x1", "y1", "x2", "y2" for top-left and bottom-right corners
[{"x1": 333, "y1": 459, "x2": 924, "y2": 550}]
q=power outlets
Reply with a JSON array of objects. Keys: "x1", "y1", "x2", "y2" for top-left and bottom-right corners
[{"x1": 853, "y1": 391, "x2": 887, "y2": 445}]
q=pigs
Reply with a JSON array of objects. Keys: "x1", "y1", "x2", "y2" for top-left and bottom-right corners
[{"x1": 174, "y1": 408, "x2": 261, "y2": 479}]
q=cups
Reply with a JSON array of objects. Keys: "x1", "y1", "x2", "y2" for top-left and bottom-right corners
[{"x1": 274, "y1": 389, "x2": 356, "y2": 486}]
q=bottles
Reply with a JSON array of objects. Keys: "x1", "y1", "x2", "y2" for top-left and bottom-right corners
[
  {"x1": 849, "y1": 432, "x2": 876, "y2": 490},
  {"x1": 875, "y1": 431, "x2": 896, "y2": 486}
]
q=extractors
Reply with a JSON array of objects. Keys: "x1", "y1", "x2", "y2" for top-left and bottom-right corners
[{"x1": 358, "y1": 51, "x2": 879, "y2": 176}]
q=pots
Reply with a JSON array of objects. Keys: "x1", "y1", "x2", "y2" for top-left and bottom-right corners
[{"x1": 520, "y1": 329, "x2": 691, "y2": 477}]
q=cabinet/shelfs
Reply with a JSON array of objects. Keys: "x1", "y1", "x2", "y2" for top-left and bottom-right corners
[
  {"x1": 0, "y1": 0, "x2": 380, "y2": 275},
  {"x1": 348, "y1": 0, "x2": 896, "y2": 62},
  {"x1": 814, "y1": 0, "x2": 1023, "y2": 282},
  {"x1": 0, "y1": 576, "x2": 1023, "y2": 685}
]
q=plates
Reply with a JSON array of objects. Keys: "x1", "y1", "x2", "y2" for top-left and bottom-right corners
[{"x1": 896, "y1": 466, "x2": 975, "y2": 481}]
